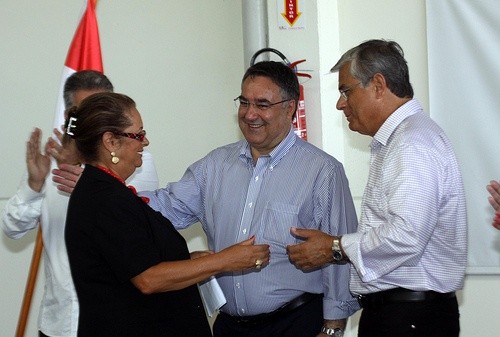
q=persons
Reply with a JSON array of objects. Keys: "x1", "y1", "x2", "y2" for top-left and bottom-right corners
[
  {"x1": 289, "y1": 37, "x2": 467, "y2": 337},
  {"x1": 63, "y1": 92, "x2": 270, "y2": 337},
  {"x1": 0, "y1": 67, "x2": 157, "y2": 337},
  {"x1": 52, "y1": 58, "x2": 358, "y2": 337}
]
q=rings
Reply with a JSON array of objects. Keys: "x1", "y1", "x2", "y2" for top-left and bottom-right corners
[{"x1": 254, "y1": 258, "x2": 262, "y2": 269}]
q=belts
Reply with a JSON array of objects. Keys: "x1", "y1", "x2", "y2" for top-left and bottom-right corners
[
  {"x1": 357, "y1": 288, "x2": 455, "y2": 309},
  {"x1": 219, "y1": 292, "x2": 316, "y2": 326}
]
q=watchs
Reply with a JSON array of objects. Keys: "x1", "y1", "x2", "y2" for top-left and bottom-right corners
[
  {"x1": 332, "y1": 234, "x2": 343, "y2": 264},
  {"x1": 322, "y1": 326, "x2": 344, "y2": 337}
]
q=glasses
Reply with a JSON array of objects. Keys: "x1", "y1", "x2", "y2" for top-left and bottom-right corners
[
  {"x1": 340, "y1": 76, "x2": 373, "y2": 102},
  {"x1": 101, "y1": 129, "x2": 146, "y2": 142},
  {"x1": 234, "y1": 96, "x2": 292, "y2": 112}
]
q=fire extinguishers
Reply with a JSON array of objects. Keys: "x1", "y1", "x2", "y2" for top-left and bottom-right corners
[{"x1": 250, "y1": 47, "x2": 311, "y2": 142}]
